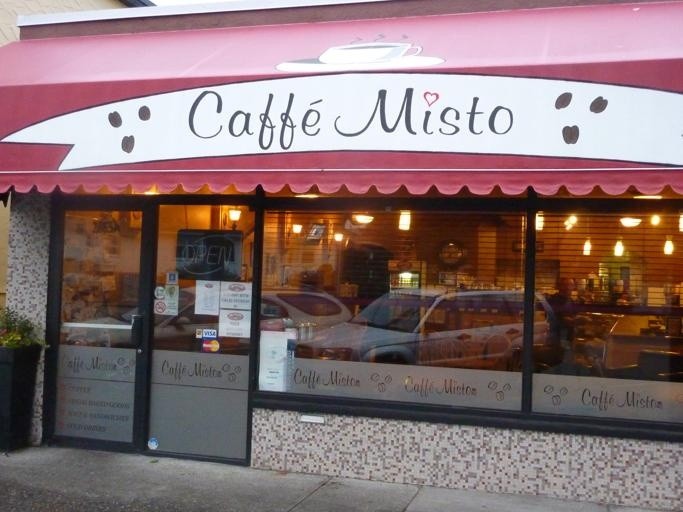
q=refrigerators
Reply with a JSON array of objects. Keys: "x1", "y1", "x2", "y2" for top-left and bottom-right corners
[{"x1": 387, "y1": 259, "x2": 426, "y2": 335}]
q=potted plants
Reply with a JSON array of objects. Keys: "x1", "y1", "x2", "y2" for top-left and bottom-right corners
[{"x1": 0, "y1": 304, "x2": 52, "y2": 451}]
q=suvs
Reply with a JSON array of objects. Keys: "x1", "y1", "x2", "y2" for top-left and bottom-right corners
[{"x1": 295, "y1": 288, "x2": 558, "y2": 372}]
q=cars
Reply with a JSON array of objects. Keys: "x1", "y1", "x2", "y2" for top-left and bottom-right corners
[{"x1": 62, "y1": 287, "x2": 352, "y2": 355}]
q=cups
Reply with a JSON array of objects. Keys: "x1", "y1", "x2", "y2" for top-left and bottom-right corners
[{"x1": 319, "y1": 42, "x2": 422, "y2": 64}]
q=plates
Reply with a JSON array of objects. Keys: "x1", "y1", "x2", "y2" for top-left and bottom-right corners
[{"x1": 275, "y1": 55, "x2": 445, "y2": 73}]
